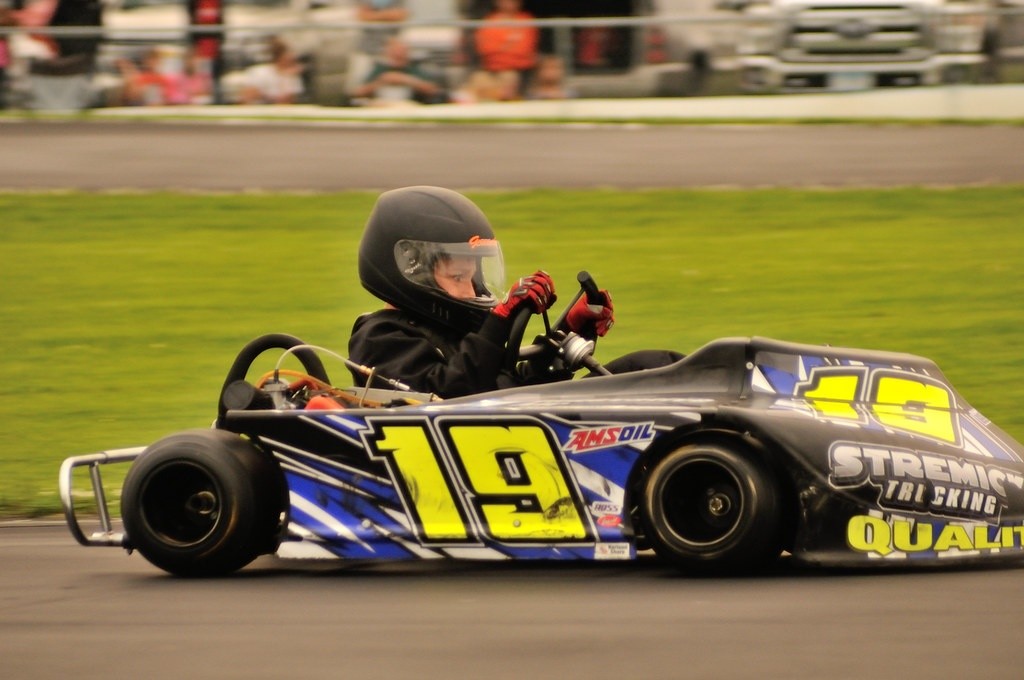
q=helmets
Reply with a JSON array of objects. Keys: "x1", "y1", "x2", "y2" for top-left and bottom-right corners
[{"x1": 357, "y1": 186, "x2": 500, "y2": 333}]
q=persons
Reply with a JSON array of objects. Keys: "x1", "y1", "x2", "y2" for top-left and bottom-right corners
[
  {"x1": 346, "y1": 186, "x2": 617, "y2": 402},
  {"x1": 0, "y1": 0, "x2": 671, "y2": 103}
]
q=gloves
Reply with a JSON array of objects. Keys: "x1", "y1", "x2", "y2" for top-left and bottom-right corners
[
  {"x1": 566, "y1": 289, "x2": 615, "y2": 337},
  {"x1": 496, "y1": 270, "x2": 555, "y2": 317}
]
